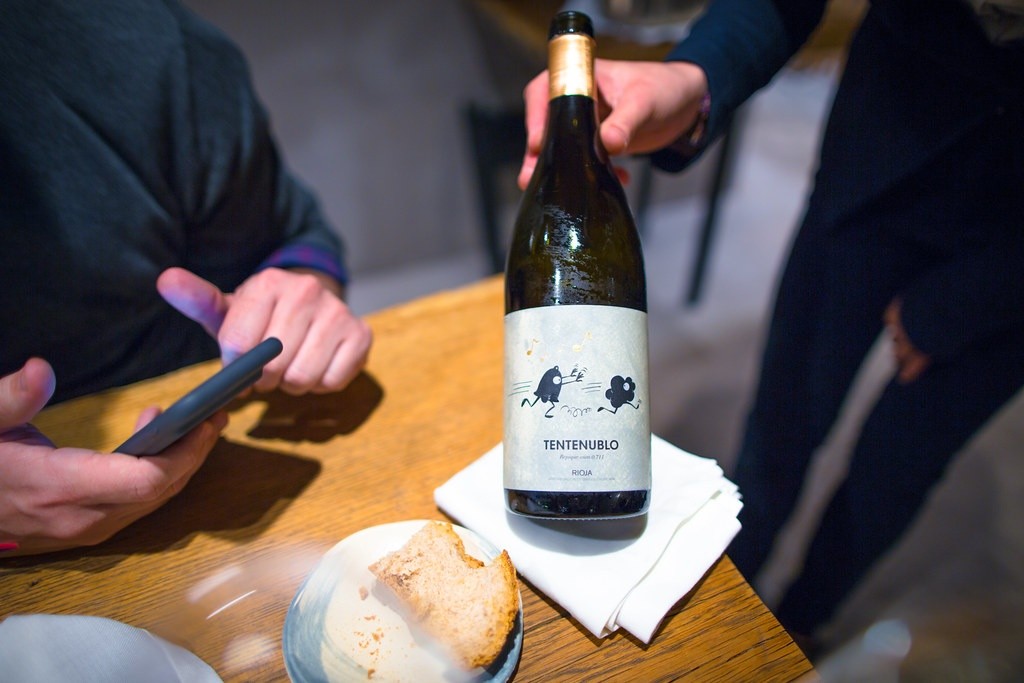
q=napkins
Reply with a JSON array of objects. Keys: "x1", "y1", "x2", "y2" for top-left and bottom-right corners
[{"x1": 435, "y1": 432, "x2": 743, "y2": 642}]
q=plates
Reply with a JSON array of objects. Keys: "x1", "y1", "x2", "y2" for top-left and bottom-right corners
[{"x1": 282, "y1": 519, "x2": 524, "y2": 683}]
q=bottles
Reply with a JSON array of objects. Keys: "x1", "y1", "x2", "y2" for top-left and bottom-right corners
[{"x1": 504, "y1": 11, "x2": 652, "y2": 525}]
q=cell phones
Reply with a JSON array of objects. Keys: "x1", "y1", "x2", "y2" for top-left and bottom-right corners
[{"x1": 110, "y1": 338, "x2": 283, "y2": 457}]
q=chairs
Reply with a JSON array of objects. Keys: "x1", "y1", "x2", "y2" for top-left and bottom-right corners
[{"x1": 454, "y1": 0, "x2": 743, "y2": 299}]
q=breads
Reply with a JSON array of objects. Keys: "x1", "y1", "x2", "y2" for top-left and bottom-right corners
[{"x1": 362, "y1": 519, "x2": 520, "y2": 672}]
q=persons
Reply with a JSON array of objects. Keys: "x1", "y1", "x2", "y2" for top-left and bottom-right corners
[
  {"x1": 516, "y1": 0, "x2": 1024, "y2": 661},
  {"x1": 0, "y1": 0, "x2": 375, "y2": 564}
]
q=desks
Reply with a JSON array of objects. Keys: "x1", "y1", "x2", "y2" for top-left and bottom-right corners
[{"x1": 0, "y1": 276, "x2": 824, "y2": 682}]
